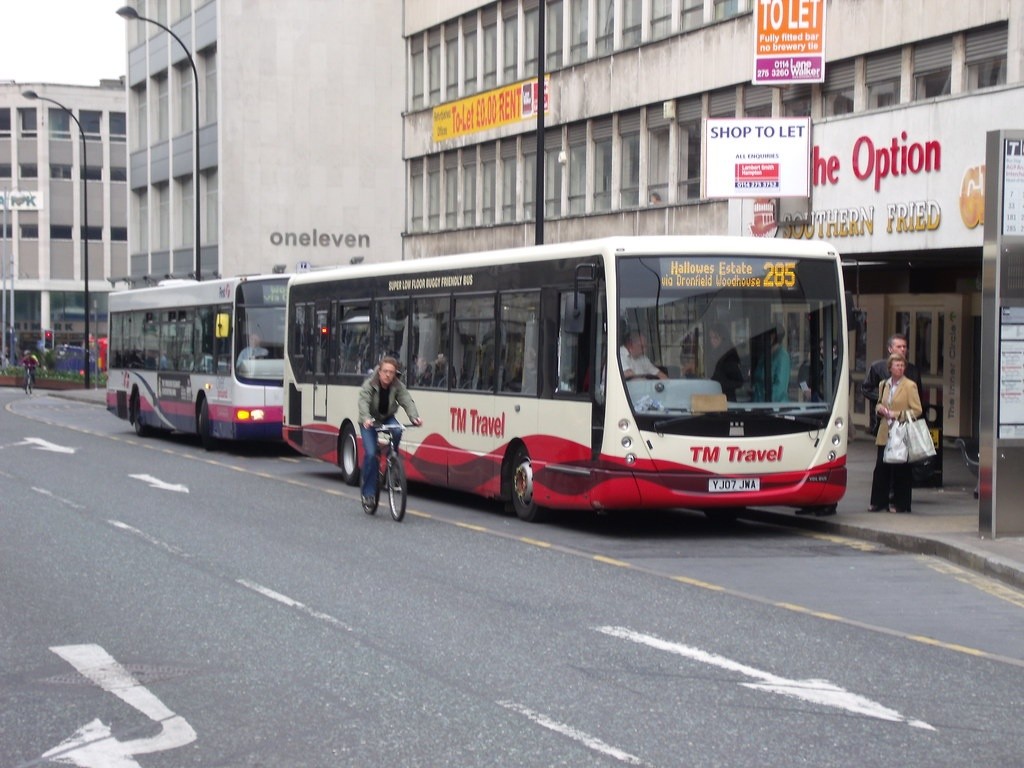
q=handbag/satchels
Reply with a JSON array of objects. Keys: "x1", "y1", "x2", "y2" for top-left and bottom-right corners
[
  {"x1": 904, "y1": 408, "x2": 936, "y2": 463},
  {"x1": 883, "y1": 410, "x2": 908, "y2": 466}
]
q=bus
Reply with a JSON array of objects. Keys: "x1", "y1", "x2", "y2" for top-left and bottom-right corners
[
  {"x1": 106, "y1": 272, "x2": 300, "y2": 447},
  {"x1": 280, "y1": 233, "x2": 864, "y2": 523}
]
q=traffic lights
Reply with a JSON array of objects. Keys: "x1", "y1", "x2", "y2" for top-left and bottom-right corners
[{"x1": 43, "y1": 329, "x2": 53, "y2": 351}]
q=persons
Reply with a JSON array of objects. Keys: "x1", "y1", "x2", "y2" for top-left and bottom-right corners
[
  {"x1": 18, "y1": 350, "x2": 40, "y2": 388},
  {"x1": 237, "y1": 331, "x2": 268, "y2": 372},
  {"x1": 602, "y1": 324, "x2": 851, "y2": 516},
  {"x1": 356, "y1": 356, "x2": 422, "y2": 509},
  {"x1": 862, "y1": 336, "x2": 928, "y2": 512}
]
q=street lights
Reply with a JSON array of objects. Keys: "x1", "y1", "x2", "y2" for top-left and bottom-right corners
[
  {"x1": 116, "y1": 4, "x2": 204, "y2": 280},
  {"x1": 20, "y1": 91, "x2": 92, "y2": 388}
]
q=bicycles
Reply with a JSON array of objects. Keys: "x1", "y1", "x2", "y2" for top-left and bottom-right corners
[
  {"x1": 359, "y1": 416, "x2": 421, "y2": 524},
  {"x1": 19, "y1": 364, "x2": 40, "y2": 395}
]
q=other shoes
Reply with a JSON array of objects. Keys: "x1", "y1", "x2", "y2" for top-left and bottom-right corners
[
  {"x1": 868, "y1": 505, "x2": 883, "y2": 512},
  {"x1": 362, "y1": 494, "x2": 378, "y2": 514},
  {"x1": 889, "y1": 505, "x2": 897, "y2": 513}
]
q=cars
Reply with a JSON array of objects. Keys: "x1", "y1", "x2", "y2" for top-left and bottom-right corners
[{"x1": 54, "y1": 335, "x2": 107, "y2": 377}]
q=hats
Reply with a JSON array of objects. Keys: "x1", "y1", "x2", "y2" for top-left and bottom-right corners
[{"x1": 24, "y1": 350, "x2": 31, "y2": 355}]
